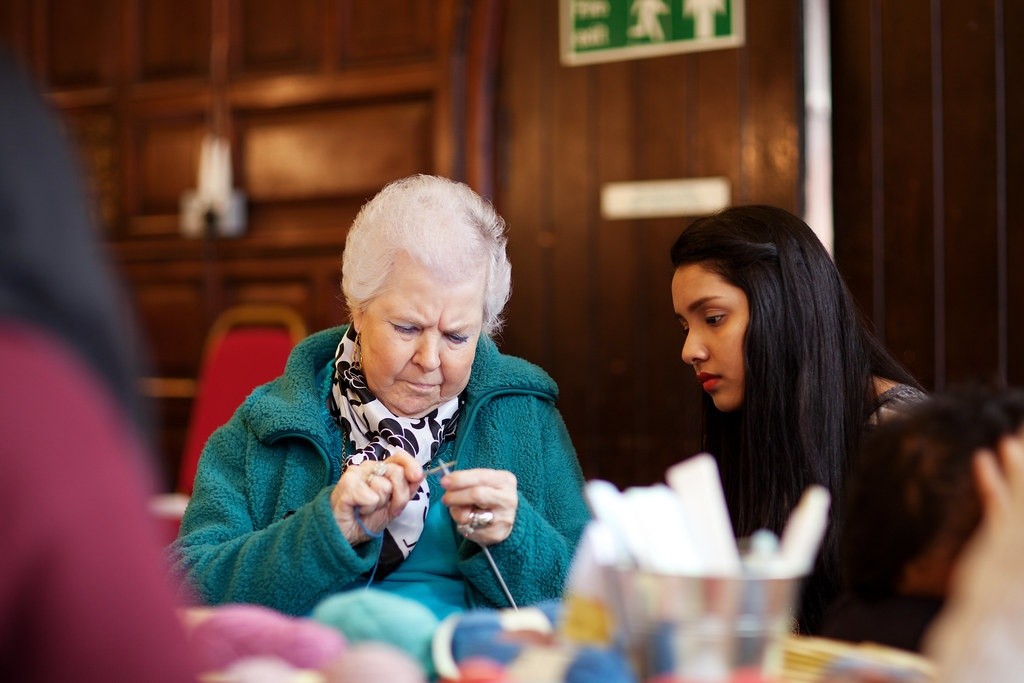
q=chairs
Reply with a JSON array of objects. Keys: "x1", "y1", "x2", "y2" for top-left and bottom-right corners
[{"x1": 149, "y1": 303, "x2": 307, "y2": 542}]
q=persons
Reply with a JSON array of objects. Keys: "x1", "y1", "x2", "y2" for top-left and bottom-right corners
[
  {"x1": 816, "y1": 392, "x2": 1024, "y2": 683},
  {"x1": 169, "y1": 176, "x2": 592, "y2": 618},
  {"x1": 0, "y1": 55, "x2": 199, "y2": 682},
  {"x1": 671, "y1": 205, "x2": 931, "y2": 628}
]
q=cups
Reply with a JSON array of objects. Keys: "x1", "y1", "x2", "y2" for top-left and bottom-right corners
[{"x1": 600, "y1": 566, "x2": 804, "y2": 683}]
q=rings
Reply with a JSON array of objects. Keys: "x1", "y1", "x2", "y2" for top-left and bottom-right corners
[
  {"x1": 375, "y1": 462, "x2": 387, "y2": 475},
  {"x1": 366, "y1": 474, "x2": 374, "y2": 484},
  {"x1": 468, "y1": 510, "x2": 493, "y2": 527}
]
